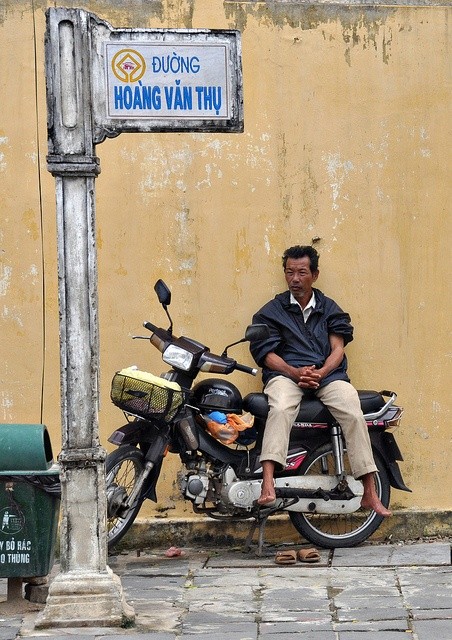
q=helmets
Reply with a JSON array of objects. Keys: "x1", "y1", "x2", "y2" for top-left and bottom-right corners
[{"x1": 189, "y1": 378, "x2": 243, "y2": 414}]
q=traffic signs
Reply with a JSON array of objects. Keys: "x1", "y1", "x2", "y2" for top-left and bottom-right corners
[{"x1": 105, "y1": 40, "x2": 231, "y2": 120}]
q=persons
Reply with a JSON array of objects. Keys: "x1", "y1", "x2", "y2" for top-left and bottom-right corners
[{"x1": 248, "y1": 244, "x2": 396, "y2": 518}]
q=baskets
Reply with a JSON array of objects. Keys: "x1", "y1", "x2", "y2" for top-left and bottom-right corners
[{"x1": 110, "y1": 371, "x2": 194, "y2": 426}]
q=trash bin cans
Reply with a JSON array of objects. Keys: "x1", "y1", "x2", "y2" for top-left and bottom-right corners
[{"x1": 0, "y1": 423, "x2": 62, "y2": 578}]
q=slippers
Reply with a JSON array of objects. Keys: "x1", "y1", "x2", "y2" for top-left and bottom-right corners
[
  {"x1": 297, "y1": 547, "x2": 321, "y2": 562},
  {"x1": 274, "y1": 548, "x2": 298, "y2": 564}
]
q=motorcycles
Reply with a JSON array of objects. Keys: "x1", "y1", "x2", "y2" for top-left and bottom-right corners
[{"x1": 103, "y1": 278, "x2": 413, "y2": 550}]
